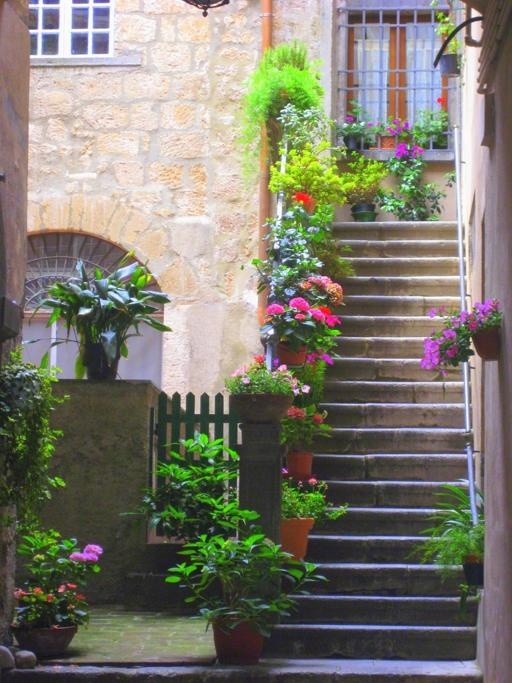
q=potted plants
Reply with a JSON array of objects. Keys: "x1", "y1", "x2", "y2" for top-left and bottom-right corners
[
  {"x1": 166, "y1": 509, "x2": 327, "y2": 663},
  {"x1": 431, "y1": 11, "x2": 463, "y2": 75},
  {"x1": 20, "y1": 249, "x2": 174, "y2": 382},
  {"x1": 404, "y1": 476, "x2": 484, "y2": 593},
  {"x1": 336, "y1": 158, "x2": 391, "y2": 223}
]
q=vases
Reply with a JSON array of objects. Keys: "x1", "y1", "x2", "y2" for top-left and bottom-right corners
[
  {"x1": 8, "y1": 628, "x2": 92, "y2": 660},
  {"x1": 282, "y1": 520, "x2": 351, "y2": 562},
  {"x1": 471, "y1": 327, "x2": 504, "y2": 362},
  {"x1": 345, "y1": 136, "x2": 372, "y2": 151},
  {"x1": 283, "y1": 343, "x2": 310, "y2": 364},
  {"x1": 283, "y1": 451, "x2": 338, "y2": 480},
  {"x1": 240, "y1": 397, "x2": 296, "y2": 424}
]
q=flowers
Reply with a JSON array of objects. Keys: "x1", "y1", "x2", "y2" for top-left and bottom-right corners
[
  {"x1": 16, "y1": 537, "x2": 104, "y2": 628},
  {"x1": 223, "y1": 352, "x2": 312, "y2": 395},
  {"x1": 276, "y1": 405, "x2": 335, "y2": 451},
  {"x1": 258, "y1": 296, "x2": 342, "y2": 352},
  {"x1": 418, "y1": 298, "x2": 503, "y2": 375},
  {"x1": 281, "y1": 465, "x2": 350, "y2": 523},
  {"x1": 330, "y1": 98, "x2": 382, "y2": 138}
]
